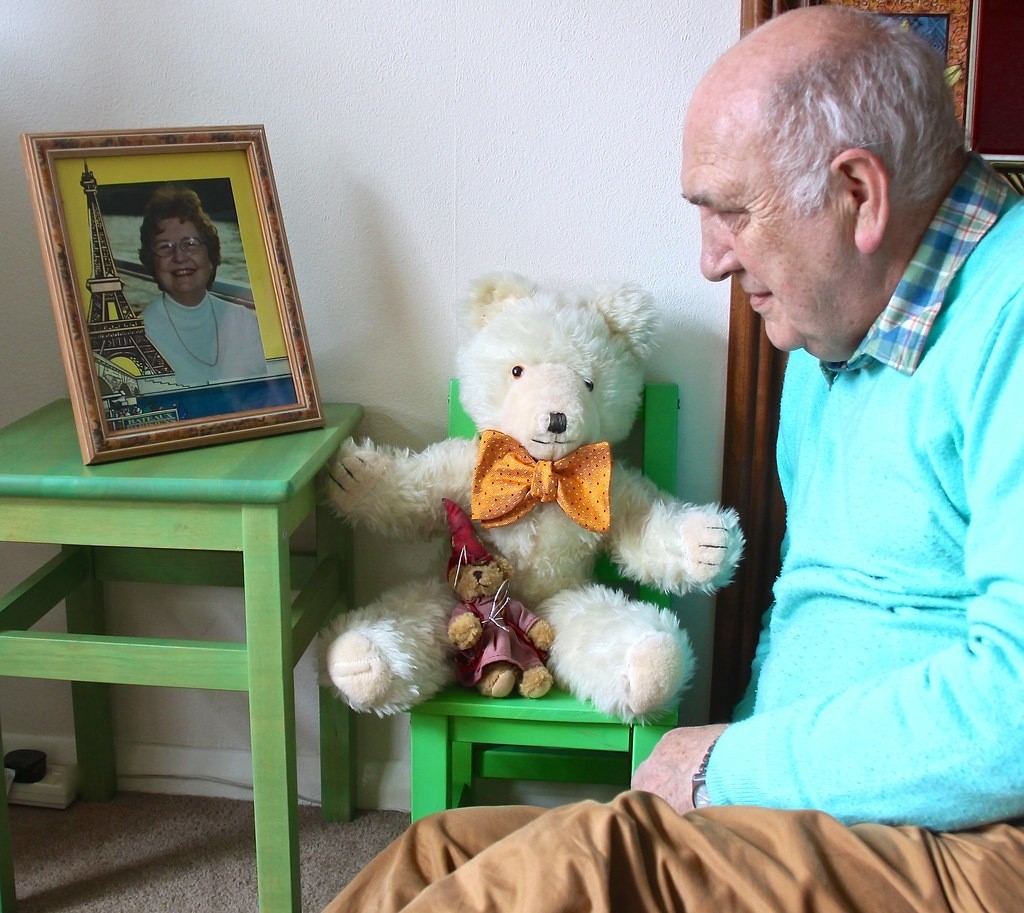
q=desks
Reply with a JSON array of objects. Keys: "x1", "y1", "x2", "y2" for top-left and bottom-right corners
[{"x1": 1, "y1": 396, "x2": 364, "y2": 913}]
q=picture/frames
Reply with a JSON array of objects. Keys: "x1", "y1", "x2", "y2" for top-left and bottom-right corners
[{"x1": 22, "y1": 124, "x2": 326, "y2": 466}]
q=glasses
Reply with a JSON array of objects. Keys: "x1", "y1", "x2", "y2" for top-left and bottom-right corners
[{"x1": 149, "y1": 237, "x2": 207, "y2": 257}]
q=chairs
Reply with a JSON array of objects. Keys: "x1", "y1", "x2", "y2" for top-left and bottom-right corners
[{"x1": 414, "y1": 382, "x2": 686, "y2": 820}]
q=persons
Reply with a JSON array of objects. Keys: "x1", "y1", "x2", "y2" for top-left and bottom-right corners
[
  {"x1": 324, "y1": 4, "x2": 1024, "y2": 913},
  {"x1": 136, "y1": 186, "x2": 267, "y2": 384}
]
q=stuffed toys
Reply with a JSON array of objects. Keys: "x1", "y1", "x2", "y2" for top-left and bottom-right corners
[
  {"x1": 441, "y1": 498, "x2": 555, "y2": 699},
  {"x1": 320, "y1": 272, "x2": 746, "y2": 726}
]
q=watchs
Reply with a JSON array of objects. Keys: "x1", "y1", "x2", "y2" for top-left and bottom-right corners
[{"x1": 691, "y1": 735, "x2": 721, "y2": 809}]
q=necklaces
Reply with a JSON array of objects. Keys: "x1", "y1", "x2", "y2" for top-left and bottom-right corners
[{"x1": 162, "y1": 289, "x2": 218, "y2": 367}]
